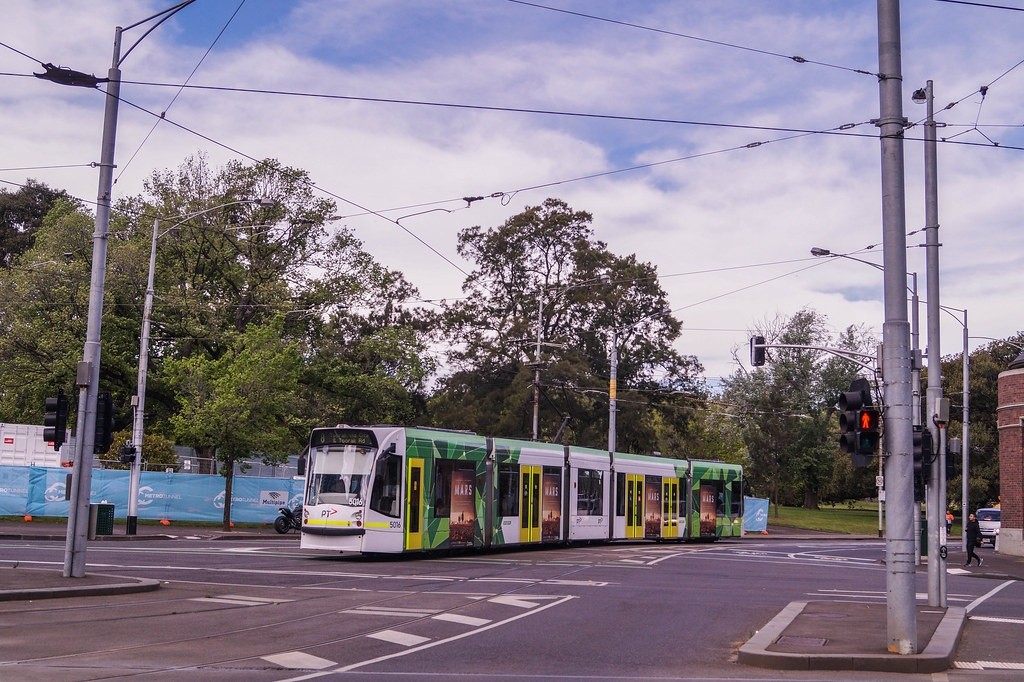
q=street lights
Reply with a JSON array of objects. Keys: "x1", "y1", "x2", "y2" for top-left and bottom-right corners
[{"x1": 809, "y1": 247, "x2": 924, "y2": 567}]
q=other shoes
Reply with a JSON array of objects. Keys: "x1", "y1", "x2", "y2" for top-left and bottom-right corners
[
  {"x1": 948, "y1": 534, "x2": 950, "y2": 536},
  {"x1": 978, "y1": 558, "x2": 983, "y2": 567},
  {"x1": 964, "y1": 563, "x2": 973, "y2": 567}
]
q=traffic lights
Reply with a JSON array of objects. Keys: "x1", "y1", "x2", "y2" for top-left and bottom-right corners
[
  {"x1": 42, "y1": 390, "x2": 69, "y2": 453},
  {"x1": 838, "y1": 378, "x2": 869, "y2": 454},
  {"x1": 856, "y1": 409, "x2": 880, "y2": 454}
]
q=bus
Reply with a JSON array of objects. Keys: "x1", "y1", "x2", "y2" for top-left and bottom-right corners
[{"x1": 298, "y1": 381, "x2": 747, "y2": 556}]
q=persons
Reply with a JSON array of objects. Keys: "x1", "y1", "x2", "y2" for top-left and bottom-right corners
[
  {"x1": 921, "y1": 501, "x2": 926, "y2": 515},
  {"x1": 945, "y1": 511, "x2": 954, "y2": 536},
  {"x1": 963, "y1": 512, "x2": 984, "y2": 568}
]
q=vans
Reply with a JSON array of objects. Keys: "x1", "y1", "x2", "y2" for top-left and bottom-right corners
[{"x1": 972, "y1": 508, "x2": 1000, "y2": 547}]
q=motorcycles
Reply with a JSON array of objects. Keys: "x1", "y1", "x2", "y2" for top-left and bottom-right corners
[{"x1": 275, "y1": 504, "x2": 303, "y2": 535}]
